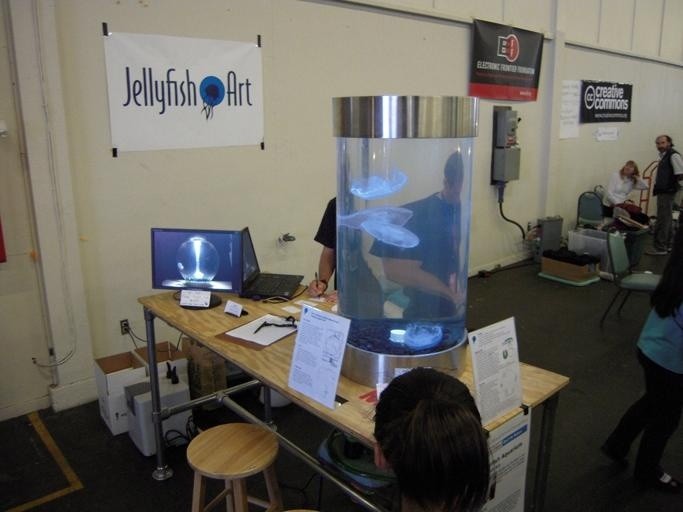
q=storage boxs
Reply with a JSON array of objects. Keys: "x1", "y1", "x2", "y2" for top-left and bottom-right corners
[
  {"x1": 540, "y1": 251, "x2": 591, "y2": 282},
  {"x1": 181, "y1": 337, "x2": 230, "y2": 414},
  {"x1": 612, "y1": 226, "x2": 649, "y2": 266},
  {"x1": 91, "y1": 341, "x2": 190, "y2": 438},
  {"x1": 568, "y1": 228, "x2": 610, "y2": 258}
]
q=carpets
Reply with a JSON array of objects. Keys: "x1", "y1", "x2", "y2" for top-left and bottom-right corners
[{"x1": 0, "y1": 413, "x2": 83, "y2": 510}]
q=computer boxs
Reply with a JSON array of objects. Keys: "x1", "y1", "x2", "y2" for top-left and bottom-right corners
[{"x1": 125, "y1": 372, "x2": 194, "y2": 457}]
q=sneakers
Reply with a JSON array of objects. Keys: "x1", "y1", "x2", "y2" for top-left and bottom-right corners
[{"x1": 645, "y1": 248, "x2": 668, "y2": 255}]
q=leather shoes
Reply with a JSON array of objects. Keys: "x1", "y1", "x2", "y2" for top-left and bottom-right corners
[{"x1": 654, "y1": 476, "x2": 681, "y2": 493}]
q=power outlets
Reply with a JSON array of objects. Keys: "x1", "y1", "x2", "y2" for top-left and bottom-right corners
[{"x1": 119, "y1": 319, "x2": 129, "y2": 335}]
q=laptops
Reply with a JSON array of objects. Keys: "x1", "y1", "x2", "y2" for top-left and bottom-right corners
[{"x1": 240, "y1": 226, "x2": 304, "y2": 299}]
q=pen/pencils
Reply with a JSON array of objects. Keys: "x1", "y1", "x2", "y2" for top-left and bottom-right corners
[{"x1": 315, "y1": 272, "x2": 320, "y2": 300}]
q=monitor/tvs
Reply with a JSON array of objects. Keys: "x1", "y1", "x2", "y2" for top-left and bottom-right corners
[{"x1": 151, "y1": 228, "x2": 242, "y2": 310}]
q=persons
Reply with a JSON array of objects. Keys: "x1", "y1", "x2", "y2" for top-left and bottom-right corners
[
  {"x1": 308, "y1": 191, "x2": 384, "y2": 315},
  {"x1": 644, "y1": 135, "x2": 683, "y2": 255},
  {"x1": 601, "y1": 160, "x2": 650, "y2": 232},
  {"x1": 599, "y1": 216, "x2": 682, "y2": 494},
  {"x1": 371, "y1": 154, "x2": 464, "y2": 318},
  {"x1": 373, "y1": 367, "x2": 492, "y2": 511}
]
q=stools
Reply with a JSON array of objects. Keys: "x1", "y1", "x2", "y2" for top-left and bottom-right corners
[{"x1": 184, "y1": 423, "x2": 284, "y2": 512}]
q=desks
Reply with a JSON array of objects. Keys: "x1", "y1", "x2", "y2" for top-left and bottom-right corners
[{"x1": 138, "y1": 269, "x2": 570, "y2": 510}]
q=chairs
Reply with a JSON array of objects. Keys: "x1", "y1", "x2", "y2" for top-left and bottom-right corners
[
  {"x1": 593, "y1": 183, "x2": 611, "y2": 217},
  {"x1": 599, "y1": 228, "x2": 661, "y2": 326},
  {"x1": 573, "y1": 191, "x2": 604, "y2": 228}
]
